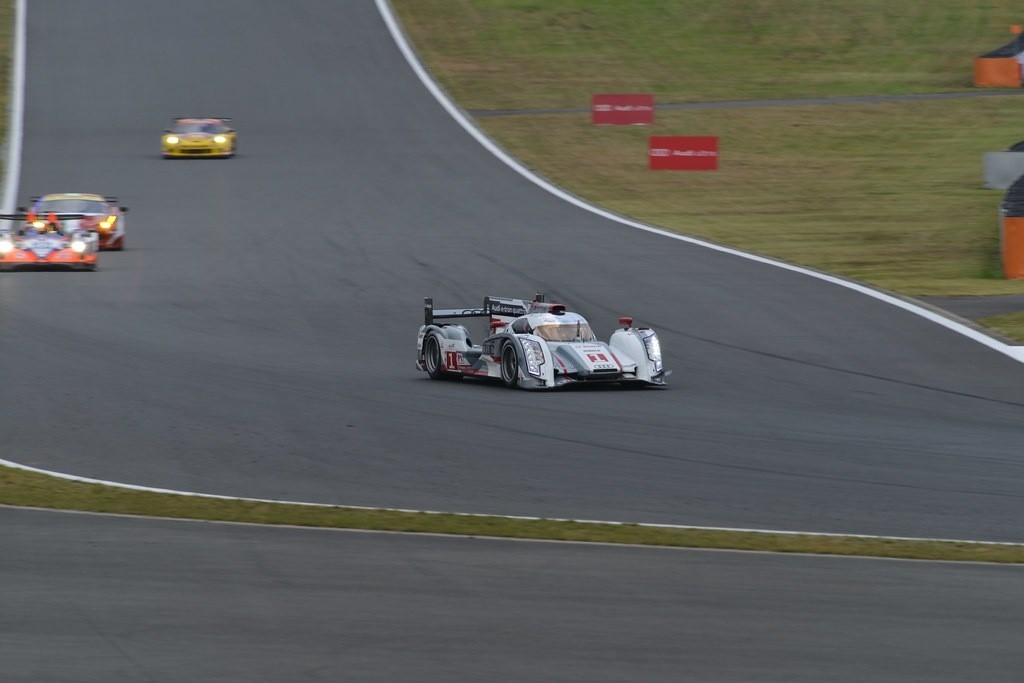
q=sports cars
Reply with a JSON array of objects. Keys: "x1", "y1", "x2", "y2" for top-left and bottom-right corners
[
  {"x1": 23, "y1": 192, "x2": 125, "y2": 250},
  {"x1": 160, "y1": 116, "x2": 238, "y2": 160}
]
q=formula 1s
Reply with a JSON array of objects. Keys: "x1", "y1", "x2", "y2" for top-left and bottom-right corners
[
  {"x1": 415, "y1": 291, "x2": 674, "y2": 391},
  {"x1": 0, "y1": 211, "x2": 101, "y2": 272}
]
